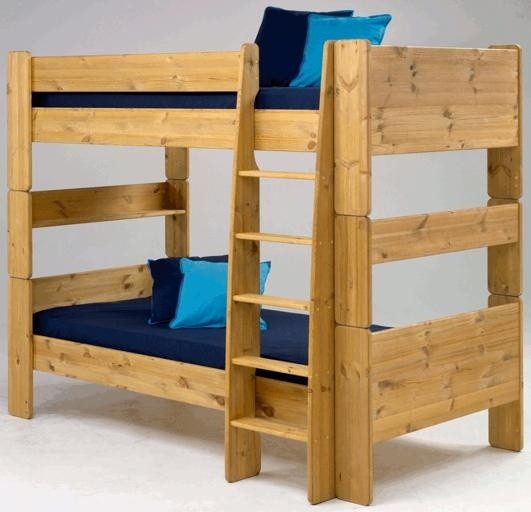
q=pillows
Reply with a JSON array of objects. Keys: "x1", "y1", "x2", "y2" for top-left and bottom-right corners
[
  {"x1": 290, "y1": 13, "x2": 391, "y2": 90},
  {"x1": 170, "y1": 259, "x2": 272, "y2": 330},
  {"x1": 249, "y1": 11, "x2": 356, "y2": 87},
  {"x1": 148, "y1": 255, "x2": 234, "y2": 324}
]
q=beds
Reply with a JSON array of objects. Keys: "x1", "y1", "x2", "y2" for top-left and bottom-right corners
[{"x1": 6, "y1": 40, "x2": 523, "y2": 506}]
